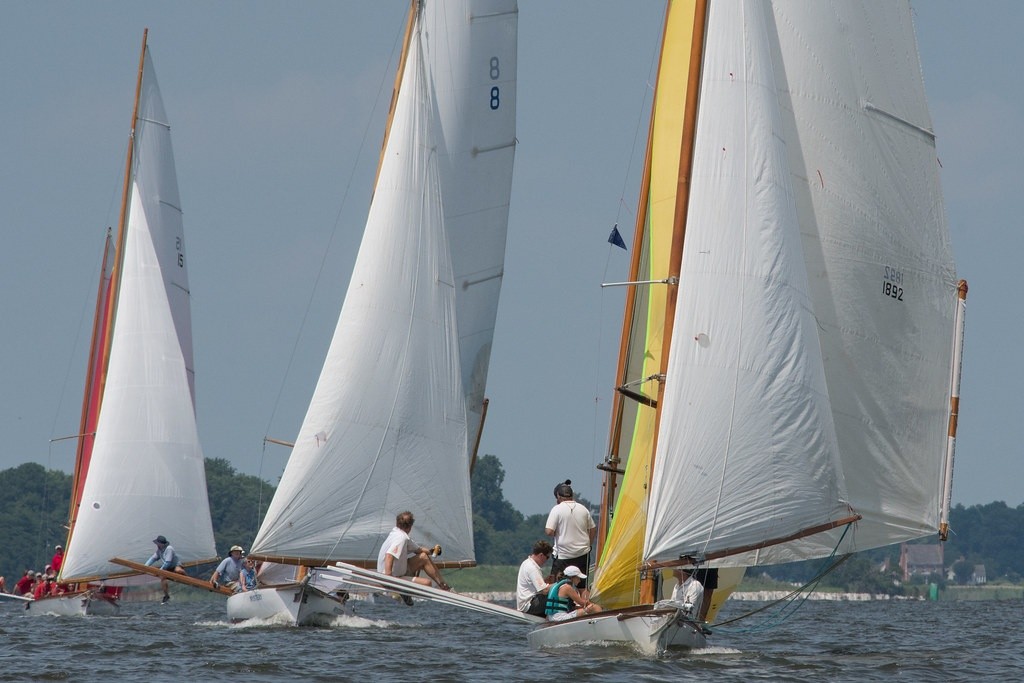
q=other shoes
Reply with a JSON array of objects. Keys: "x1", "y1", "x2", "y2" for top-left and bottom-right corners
[{"x1": 400, "y1": 594, "x2": 414, "y2": 606}]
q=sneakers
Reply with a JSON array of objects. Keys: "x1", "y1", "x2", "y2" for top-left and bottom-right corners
[{"x1": 161, "y1": 595, "x2": 170, "y2": 605}]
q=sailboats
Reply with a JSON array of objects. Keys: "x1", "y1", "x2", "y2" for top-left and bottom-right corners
[
  {"x1": 21, "y1": 28, "x2": 220, "y2": 618},
  {"x1": 527, "y1": 0, "x2": 969, "y2": 651},
  {"x1": 227, "y1": 1, "x2": 520, "y2": 624}
]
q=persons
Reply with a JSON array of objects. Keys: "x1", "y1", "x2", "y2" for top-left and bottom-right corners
[
  {"x1": 655, "y1": 567, "x2": 705, "y2": 622},
  {"x1": 0, "y1": 544, "x2": 75, "y2": 600},
  {"x1": 144, "y1": 535, "x2": 187, "y2": 605},
  {"x1": 240, "y1": 557, "x2": 265, "y2": 592},
  {"x1": 209, "y1": 545, "x2": 257, "y2": 595},
  {"x1": 545, "y1": 565, "x2": 603, "y2": 623},
  {"x1": 377, "y1": 511, "x2": 458, "y2": 606},
  {"x1": 543, "y1": 479, "x2": 596, "y2": 602},
  {"x1": 517, "y1": 539, "x2": 557, "y2": 619},
  {"x1": 684, "y1": 567, "x2": 718, "y2": 635}
]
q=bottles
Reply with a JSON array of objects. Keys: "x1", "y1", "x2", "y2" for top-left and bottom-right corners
[{"x1": 432, "y1": 544, "x2": 440, "y2": 558}]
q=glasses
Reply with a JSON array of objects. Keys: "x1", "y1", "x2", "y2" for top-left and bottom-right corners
[{"x1": 545, "y1": 554, "x2": 550, "y2": 559}]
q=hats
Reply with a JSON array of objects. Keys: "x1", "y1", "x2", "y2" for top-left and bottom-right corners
[
  {"x1": 45, "y1": 565, "x2": 51, "y2": 572},
  {"x1": 28, "y1": 570, "x2": 34, "y2": 576},
  {"x1": 55, "y1": 545, "x2": 62, "y2": 549},
  {"x1": 36, "y1": 572, "x2": 41, "y2": 577},
  {"x1": 563, "y1": 565, "x2": 587, "y2": 578},
  {"x1": 228, "y1": 545, "x2": 243, "y2": 556},
  {"x1": 152, "y1": 535, "x2": 169, "y2": 546}
]
